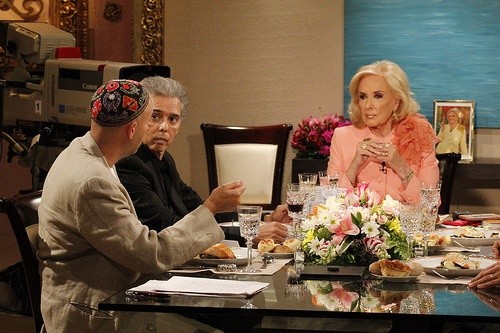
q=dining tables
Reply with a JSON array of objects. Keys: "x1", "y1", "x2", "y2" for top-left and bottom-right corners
[{"x1": 92, "y1": 222, "x2": 500, "y2": 333}]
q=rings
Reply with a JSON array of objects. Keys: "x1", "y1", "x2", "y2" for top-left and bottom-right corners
[
  {"x1": 384, "y1": 142, "x2": 392, "y2": 148},
  {"x1": 490, "y1": 298, "x2": 494, "y2": 303},
  {"x1": 490, "y1": 274, "x2": 494, "y2": 281},
  {"x1": 360, "y1": 143, "x2": 368, "y2": 151},
  {"x1": 382, "y1": 151, "x2": 388, "y2": 157}
]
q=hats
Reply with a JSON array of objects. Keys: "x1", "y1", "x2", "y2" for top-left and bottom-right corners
[{"x1": 90, "y1": 79, "x2": 149, "y2": 127}]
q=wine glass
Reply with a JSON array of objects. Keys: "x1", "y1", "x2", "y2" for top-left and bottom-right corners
[
  {"x1": 298, "y1": 172, "x2": 317, "y2": 215},
  {"x1": 285, "y1": 182, "x2": 312, "y2": 242},
  {"x1": 236, "y1": 205, "x2": 262, "y2": 273}
]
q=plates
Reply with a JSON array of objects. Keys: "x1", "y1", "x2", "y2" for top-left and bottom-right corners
[
  {"x1": 458, "y1": 213, "x2": 500, "y2": 221},
  {"x1": 368, "y1": 259, "x2": 423, "y2": 282},
  {"x1": 259, "y1": 249, "x2": 294, "y2": 260},
  {"x1": 413, "y1": 256, "x2": 498, "y2": 277},
  {"x1": 193, "y1": 246, "x2": 260, "y2": 266},
  {"x1": 443, "y1": 228, "x2": 500, "y2": 246}
]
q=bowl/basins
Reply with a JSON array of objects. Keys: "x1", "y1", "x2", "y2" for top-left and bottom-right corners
[{"x1": 220, "y1": 221, "x2": 241, "y2": 239}]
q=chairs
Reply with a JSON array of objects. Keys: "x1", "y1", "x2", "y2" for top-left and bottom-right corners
[
  {"x1": 435, "y1": 152, "x2": 463, "y2": 215},
  {"x1": 199, "y1": 122, "x2": 293, "y2": 210},
  {"x1": 0, "y1": 190, "x2": 42, "y2": 333}
]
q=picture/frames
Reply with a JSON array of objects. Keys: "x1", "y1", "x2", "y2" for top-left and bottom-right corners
[{"x1": 431, "y1": 97, "x2": 479, "y2": 164}]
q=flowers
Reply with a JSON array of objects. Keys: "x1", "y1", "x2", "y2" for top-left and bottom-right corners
[
  {"x1": 297, "y1": 178, "x2": 415, "y2": 266},
  {"x1": 291, "y1": 113, "x2": 350, "y2": 155}
]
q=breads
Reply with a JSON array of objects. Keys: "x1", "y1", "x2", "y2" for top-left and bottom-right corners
[
  {"x1": 369, "y1": 259, "x2": 424, "y2": 278},
  {"x1": 258, "y1": 238, "x2": 301, "y2": 254},
  {"x1": 199, "y1": 244, "x2": 236, "y2": 260},
  {"x1": 427, "y1": 234, "x2": 451, "y2": 246},
  {"x1": 453, "y1": 225, "x2": 486, "y2": 239},
  {"x1": 444, "y1": 252, "x2": 481, "y2": 270},
  {"x1": 368, "y1": 290, "x2": 413, "y2": 306}
]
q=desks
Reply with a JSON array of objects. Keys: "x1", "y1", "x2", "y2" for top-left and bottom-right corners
[{"x1": 290, "y1": 157, "x2": 500, "y2": 219}]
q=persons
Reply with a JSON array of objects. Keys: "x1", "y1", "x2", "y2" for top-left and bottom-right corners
[
  {"x1": 467, "y1": 238, "x2": 500, "y2": 288},
  {"x1": 327, "y1": 60, "x2": 441, "y2": 223},
  {"x1": 35, "y1": 80, "x2": 245, "y2": 333},
  {"x1": 435, "y1": 108, "x2": 468, "y2": 154},
  {"x1": 467, "y1": 286, "x2": 500, "y2": 312},
  {"x1": 115, "y1": 77, "x2": 294, "y2": 248}
]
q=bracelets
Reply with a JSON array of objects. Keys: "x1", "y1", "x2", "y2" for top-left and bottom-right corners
[
  {"x1": 401, "y1": 169, "x2": 415, "y2": 181},
  {"x1": 351, "y1": 181, "x2": 358, "y2": 184}
]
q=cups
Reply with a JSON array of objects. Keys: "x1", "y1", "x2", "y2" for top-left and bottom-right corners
[
  {"x1": 419, "y1": 178, "x2": 441, "y2": 237},
  {"x1": 399, "y1": 201, "x2": 422, "y2": 245},
  {"x1": 318, "y1": 171, "x2": 339, "y2": 200}
]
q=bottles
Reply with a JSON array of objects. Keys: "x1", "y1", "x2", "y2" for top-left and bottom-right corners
[{"x1": 294, "y1": 246, "x2": 305, "y2": 272}]
